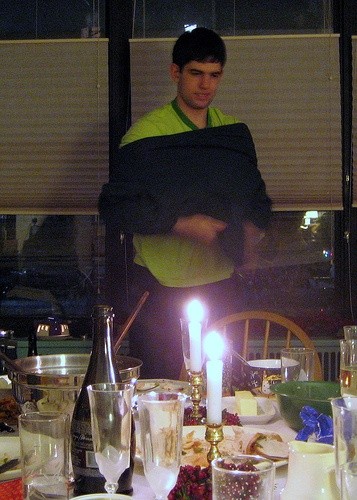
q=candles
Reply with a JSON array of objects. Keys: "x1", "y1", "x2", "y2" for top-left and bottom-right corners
[
  {"x1": 203, "y1": 331, "x2": 224, "y2": 427},
  {"x1": 187, "y1": 300, "x2": 204, "y2": 372}
]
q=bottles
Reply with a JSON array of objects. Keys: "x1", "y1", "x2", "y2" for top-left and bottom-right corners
[
  {"x1": 27, "y1": 333, "x2": 38, "y2": 357},
  {"x1": 71, "y1": 305, "x2": 134, "y2": 497}
]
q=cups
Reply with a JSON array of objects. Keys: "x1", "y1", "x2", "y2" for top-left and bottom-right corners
[
  {"x1": 280, "y1": 347, "x2": 314, "y2": 383},
  {"x1": 211, "y1": 455, "x2": 275, "y2": 500},
  {"x1": 279, "y1": 440, "x2": 344, "y2": 499},
  {"x1": 18, "y1": 411, "x2": 72, "y2": 500},
  {"x1": 331, "y1": 324, "x2": 357, "y2": 500}
]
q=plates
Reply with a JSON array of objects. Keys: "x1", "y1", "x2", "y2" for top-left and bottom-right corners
[
  {"x1": 132, "y1": 425, "x2": 291, "y2": 470},
  {"x1": 135, "y1": 378, "x2": 191, "y2": 395},
  {"x1": 0, "y1": 435, "x2": 55, "y2": 480},
  {"x1": 221, "y1": 396, "x2": 276, "y2": 424}
]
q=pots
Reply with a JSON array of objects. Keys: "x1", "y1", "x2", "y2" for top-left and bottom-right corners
[{"x1": 6, "y1": 354, "x2": 143, "y2": 409}]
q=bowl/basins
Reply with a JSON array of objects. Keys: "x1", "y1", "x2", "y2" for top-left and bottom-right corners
[
  {"x1": 269, "y1": 380, "x2": 341, "y2": 432},
  {"x1": 246, "y1": 359, "x2": 300, "y2": 398}
]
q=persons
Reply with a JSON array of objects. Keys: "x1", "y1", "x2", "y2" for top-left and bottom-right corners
[{"x1": 119, "y1": 27, "x2": 272, "y2": 381}]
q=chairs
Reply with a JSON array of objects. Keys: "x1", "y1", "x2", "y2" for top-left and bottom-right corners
[{"x1": 178, "y1": 310, "x2": 323, "y2": 384}]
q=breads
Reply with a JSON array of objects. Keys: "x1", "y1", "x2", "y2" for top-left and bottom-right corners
[{"x1": 182, "y1": 425, "x2": 288, "y2": 466}]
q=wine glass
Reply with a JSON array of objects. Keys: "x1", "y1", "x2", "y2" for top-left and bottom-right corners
[
  {"x1": 87, "y1": 382, "x2": 134, "y2": 500},
  {"x1": 135, "y1": 392, "x2": 184, "y2": 500}
]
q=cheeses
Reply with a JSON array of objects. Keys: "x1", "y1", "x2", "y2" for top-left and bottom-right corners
[{"x1": 235, "y1": 390, "x2": 258, "y2": 416}]
